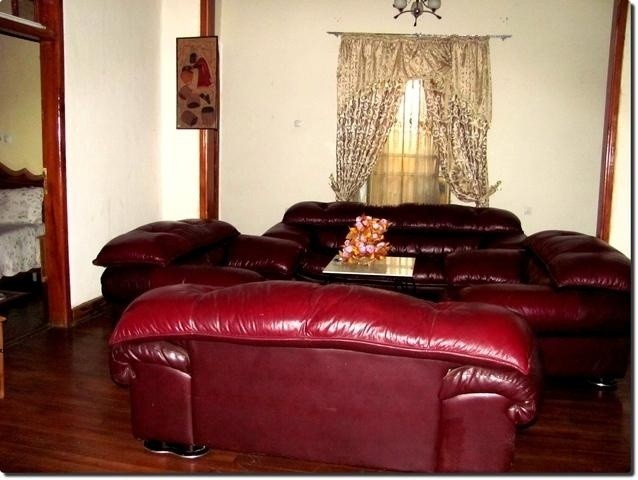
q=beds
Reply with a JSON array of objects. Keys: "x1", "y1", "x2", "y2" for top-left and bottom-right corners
[{"x1": 0, "y1": 163, "x2": 44, "y2": 280}]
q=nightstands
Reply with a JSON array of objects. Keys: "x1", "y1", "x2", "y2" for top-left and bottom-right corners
[{"x1": 36, "y1": 233, "x2": 47, "y2": 282}]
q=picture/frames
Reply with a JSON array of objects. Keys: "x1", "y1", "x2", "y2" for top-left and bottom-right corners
[{"x1": 175, "y1": 35, "x2": 219, "y2": 131}]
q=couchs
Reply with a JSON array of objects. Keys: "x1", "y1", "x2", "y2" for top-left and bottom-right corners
[
  {"x1": 264, "y1": 200, "x2": 523, "y2": 284},
  {"x1": 92, "y1": 213, "x2": 298, "y2": 317},
  {"x1": 439, "y1": 229, "x2": 630, "y2": 391},
  {"x1": 112, "y1": 282, "x2": 541, "y2": 475}
]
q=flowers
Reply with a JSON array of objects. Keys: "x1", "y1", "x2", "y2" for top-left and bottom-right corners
[{"x1": 333, "y1": 214, "x2": 395, "y2": 261}]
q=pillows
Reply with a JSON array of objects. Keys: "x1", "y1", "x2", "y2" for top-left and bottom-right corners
[{"x1": 0, "y1": 186, "x2": 44, "y2": 224}]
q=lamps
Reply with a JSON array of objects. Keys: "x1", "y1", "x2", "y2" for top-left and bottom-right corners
[{"x1": 392, "y1": 0, "x2": 442, "y2": 26}]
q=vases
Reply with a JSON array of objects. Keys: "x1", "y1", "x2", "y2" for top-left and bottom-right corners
[{"x1": 351, "y1": 255, "x2": 375, "y2": 266}]
